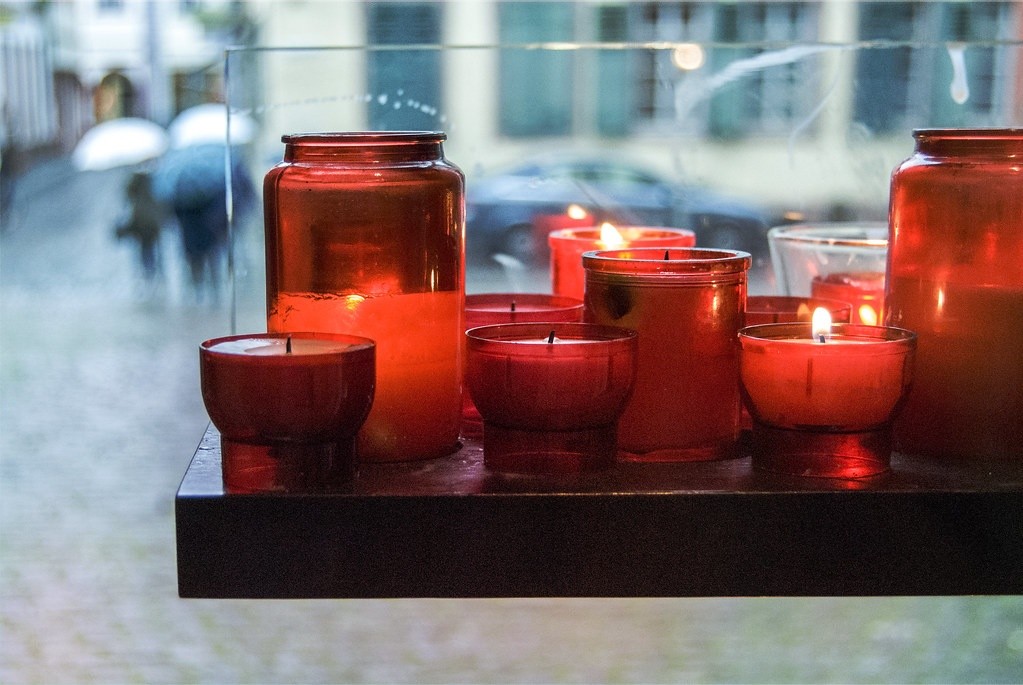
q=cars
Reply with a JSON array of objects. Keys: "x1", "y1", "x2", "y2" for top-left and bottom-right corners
[{"x1": 461, "y1": 156, "x2": 774, "y2": 264}]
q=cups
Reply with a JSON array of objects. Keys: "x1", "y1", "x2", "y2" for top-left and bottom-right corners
[{"x1": 768, "y1": 222, "x2": 888, "y2": 325}]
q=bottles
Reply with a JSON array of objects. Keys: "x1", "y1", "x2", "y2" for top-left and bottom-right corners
[
  {"x1": 884, "y1": 128, "x2": 1023, "y2": 458},
  {"x1": 263, "y1": 131, "x2": 465, "y2": 462}
]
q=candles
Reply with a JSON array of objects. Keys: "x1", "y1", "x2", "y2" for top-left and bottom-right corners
[{"x1": 200, "y1": 125, "x2": 1023, "y2": 495}]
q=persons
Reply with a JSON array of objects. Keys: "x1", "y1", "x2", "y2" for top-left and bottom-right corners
[{"x1": 122, "y1": 159, "x2": 263, "y2": 304}]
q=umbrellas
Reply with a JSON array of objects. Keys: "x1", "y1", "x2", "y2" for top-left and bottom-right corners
[
  {"x1": 168, "y1": 103, "x2": 260, "y2": 159},
  {"x1": 66, "y1": 117, "x2": 168, "y2": 176}
]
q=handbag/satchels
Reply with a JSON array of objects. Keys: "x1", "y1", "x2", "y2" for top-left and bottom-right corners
[{"x1": 115, "y1": 217, "x2": 133, "y2": 242}]
